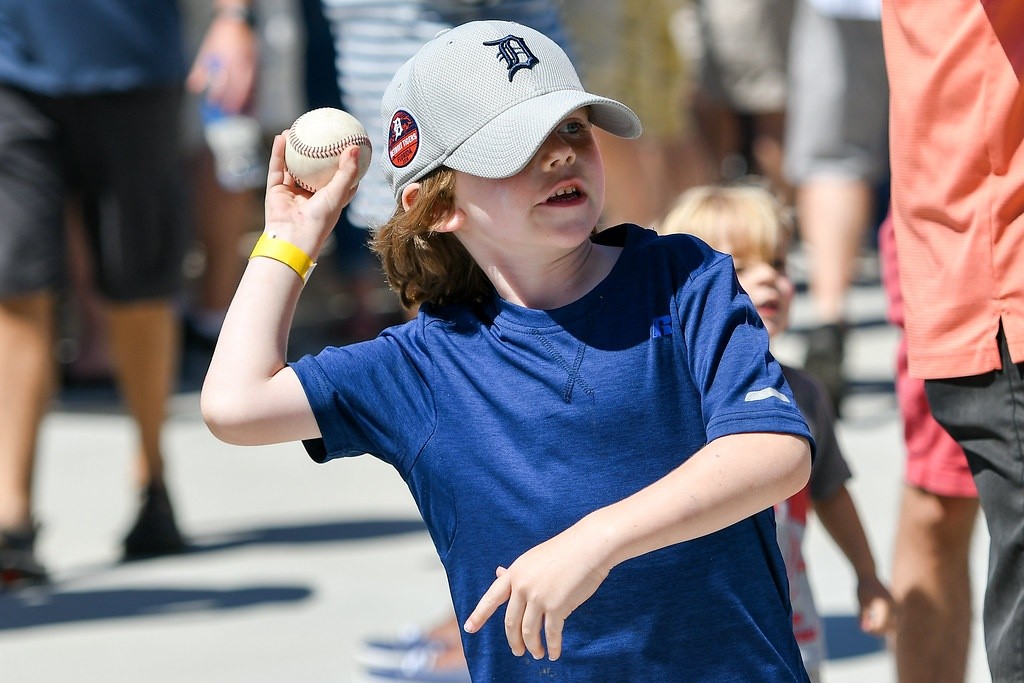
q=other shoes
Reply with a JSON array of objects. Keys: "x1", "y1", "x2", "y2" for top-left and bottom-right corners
[
  {"x1": 122, "y1": 483, "x2": 183, "y2": 558},
  {"x1": 0, "y1": 529, "x2": 49, "y2": 595}
]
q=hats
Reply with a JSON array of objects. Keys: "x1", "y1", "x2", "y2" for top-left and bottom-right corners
[{"x1": 380, "y1": 19, "x2": 643, "y2": 204}]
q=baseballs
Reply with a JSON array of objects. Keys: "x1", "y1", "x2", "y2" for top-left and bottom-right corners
[{"x1": 286, "y1": 107, "x2": 375, "y2": 197}]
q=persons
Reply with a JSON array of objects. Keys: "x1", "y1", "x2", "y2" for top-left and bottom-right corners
[
  {"x1": 878, "y1": 0, "x2": 1024, "y2": 683},
  {"x1": 200, "y1": 18, "x2": 818, "y2": 683},
  {"x1": 878, "y1": 206, "x2": 978, "y2": 683},
  {"x1": 656, "y1": 179, "x2": 904, "y2": 683},
  {"x1": 0, "y1": 0, "x2": 886, "y2": 579}
]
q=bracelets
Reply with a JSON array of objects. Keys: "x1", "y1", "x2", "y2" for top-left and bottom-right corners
[{"x1": 250, "y1": 231, "x2": 316, "y2": 286}]
